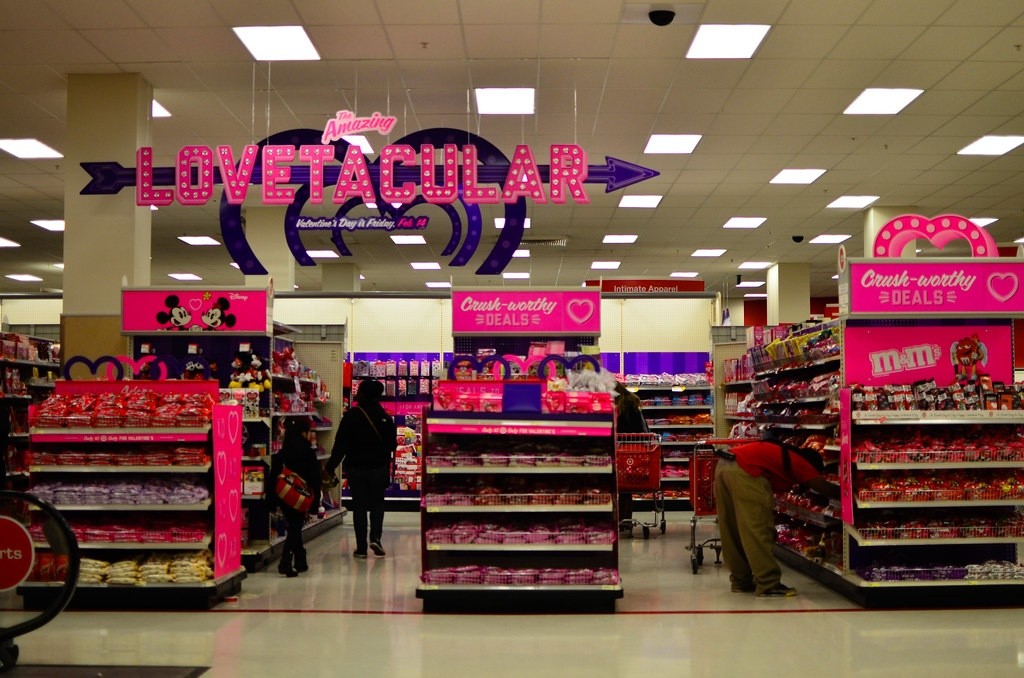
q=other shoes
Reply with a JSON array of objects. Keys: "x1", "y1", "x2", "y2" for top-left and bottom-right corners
[
  {"x1": 369, "y1": 538, "x2": 385, "y2": 556},
  {"x1": 353, "y1": 546, "x2": 367, "y2": 558}
]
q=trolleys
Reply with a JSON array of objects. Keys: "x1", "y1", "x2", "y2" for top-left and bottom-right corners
[
  {"x1": 685, "y1": 437, "x2": 758, "y2": 575},
  {"x1": 616, "y1": 431, "x2": 667, "y2": 541}
]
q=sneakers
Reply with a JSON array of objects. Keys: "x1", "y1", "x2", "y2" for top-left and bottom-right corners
[
  {"x1": 755, "y1": 581, "x2": 798, "y2": 600},
  {"x1": 731, "y1": 575, "x2": 757, "y2": 593}
]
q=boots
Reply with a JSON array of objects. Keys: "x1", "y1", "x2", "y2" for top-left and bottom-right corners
[
  {"x1": 278, "y1": 552, "x2": 298, "y2": 578},
  {"x1": 294, "y1": 548, "x2": 308, "y2": 574}
]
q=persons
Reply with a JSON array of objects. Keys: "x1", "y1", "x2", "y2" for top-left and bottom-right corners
[
  {"x1": 712, "y1": 438, "x2": 830, "y2": 597},
  {"x1": 325, "y1": 381, "x2": 399, "y2": 558},
  {"x1": 264, "y1": 417, "x2": 322, "y2": 577},
  {"x1": 614, "y1": 380, "x2": 650, "y2": 529}
]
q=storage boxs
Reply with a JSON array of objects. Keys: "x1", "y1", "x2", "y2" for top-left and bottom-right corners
[
  {"x1": 217, "y1": 388, "x2": 232, "y2": 408},
  {"x1": 243, "y1": 466, "x2": 264, "y2": 495},
  {"x1": 231, "y1": 388, "x2": 259, "y2": 419}
]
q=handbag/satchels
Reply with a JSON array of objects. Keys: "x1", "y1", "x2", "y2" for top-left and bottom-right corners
[{"x1": 273, "y1": 469, "x2": 315, "y2": 513}]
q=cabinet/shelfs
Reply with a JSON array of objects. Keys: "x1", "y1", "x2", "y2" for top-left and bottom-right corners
[
  {"x1": 122, "y1": 329, "x2": 349, "y2": 573},
  {"x1": 414, "y1": 404, "x2": 625, "y2": 614},
  {"x1": 624, "y1": 381, "x2": 712, "y2": 511},
  {"x1": 713, "y1": 313, "x2": 1024, "y2": 610},
  {"x1": 0, "y1": 330, "x2": 62, "y2": 529},
  {"x1": 24, "y1": 379, "x2": 248, "y2": 612}
]
